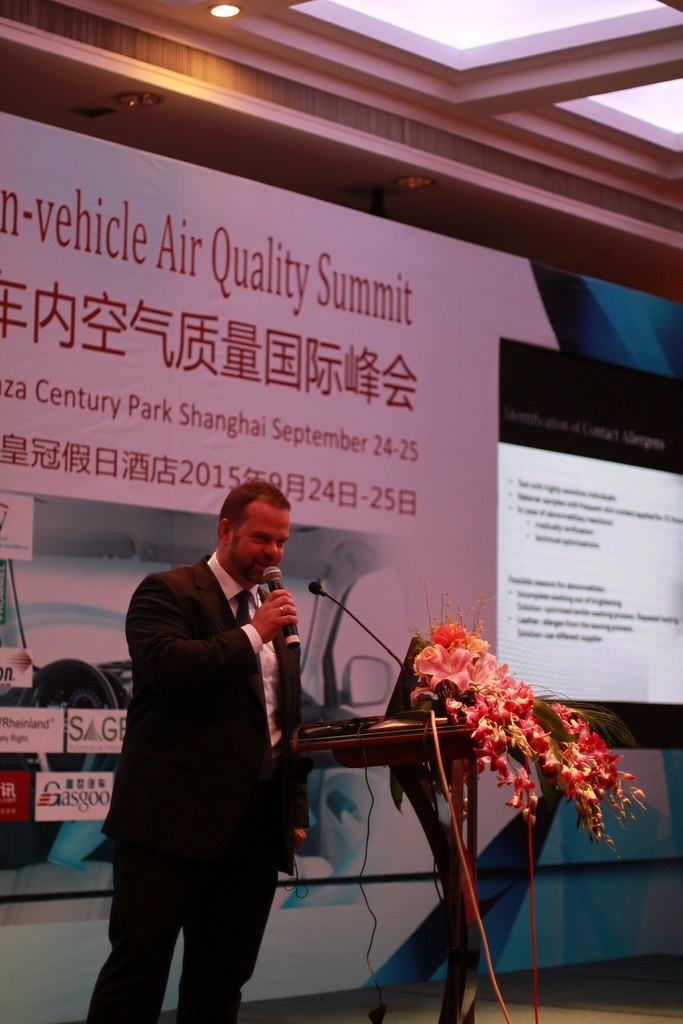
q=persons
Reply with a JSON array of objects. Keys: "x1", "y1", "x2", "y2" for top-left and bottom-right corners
[{"x1": 84, "y1": 481, "x2": 311, "y2": 1024}]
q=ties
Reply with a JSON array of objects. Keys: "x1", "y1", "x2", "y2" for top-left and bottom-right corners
[{"x1": 233, "y1": 589, "x2": 262, "y2": 676}]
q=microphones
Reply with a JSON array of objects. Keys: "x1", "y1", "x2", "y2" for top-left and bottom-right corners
[
  {"x1": 306, "y1": 581, "x2": 424, "y2": 732},
  {"x1": 263, "y1": 567, "x2": 301, "y2": 650}
]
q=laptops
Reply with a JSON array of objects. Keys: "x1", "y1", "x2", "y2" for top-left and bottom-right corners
[{"x1": 298, "y1": 638, "x2": 432, "y2": 738}]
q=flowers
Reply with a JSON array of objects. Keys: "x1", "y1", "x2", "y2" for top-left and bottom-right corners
[{"x1": 388, "y1": 580, "x2": 650, "y2": 866}]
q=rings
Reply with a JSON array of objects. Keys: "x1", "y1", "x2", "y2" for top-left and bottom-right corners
[{"x1": 280, "y1": 607, "x2": 284, "y2": 615}]
